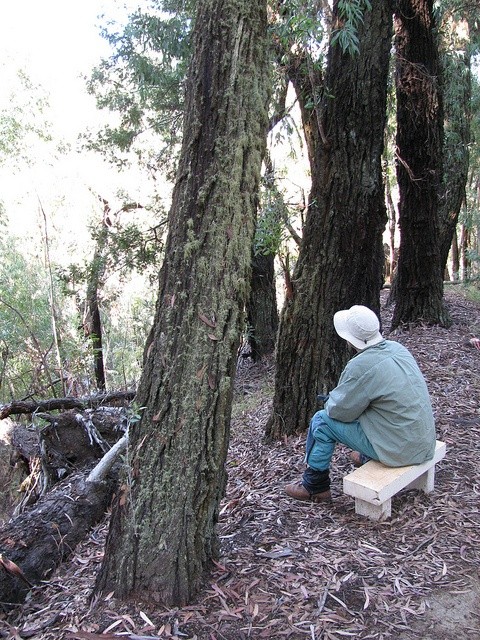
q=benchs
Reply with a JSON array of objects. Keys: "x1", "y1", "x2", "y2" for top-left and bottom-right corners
[{"x1": 342, "y1": 438, "x2": 446, "y2": 520}]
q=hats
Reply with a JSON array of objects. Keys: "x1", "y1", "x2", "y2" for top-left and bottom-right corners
[{"x1": 333, "y1": 305, "x2": 384, "y2": 349}]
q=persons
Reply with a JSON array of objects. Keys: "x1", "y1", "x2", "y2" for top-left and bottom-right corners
[{"x1": 285, "y1": 304, "x2": 436, "y2": 506}]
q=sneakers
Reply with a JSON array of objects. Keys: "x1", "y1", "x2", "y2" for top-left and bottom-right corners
[
  {"x1": 285, "y1": 480, "x2": 331, "y2": 502},
  {"x1": 351, "y1": 451, "x2": 362, "y2": 466}
]
